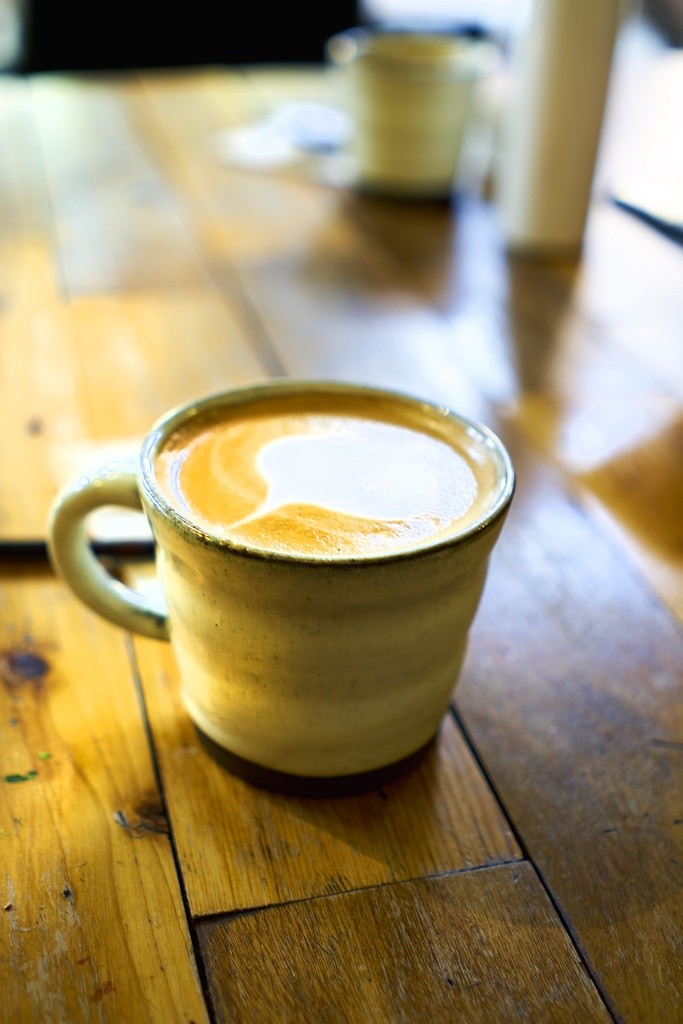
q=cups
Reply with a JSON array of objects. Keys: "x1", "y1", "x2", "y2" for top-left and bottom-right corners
[
  {"x1": 332, "y1": 18, "x2": 486, "y2": 205},
  {"x1": 48, "y1": 376, "x2": 514, "y2": 797}
]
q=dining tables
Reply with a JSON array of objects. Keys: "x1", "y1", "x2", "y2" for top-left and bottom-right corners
[{"x1": 0, "y1": 67, "x2": 683, "y2": 1024}]
